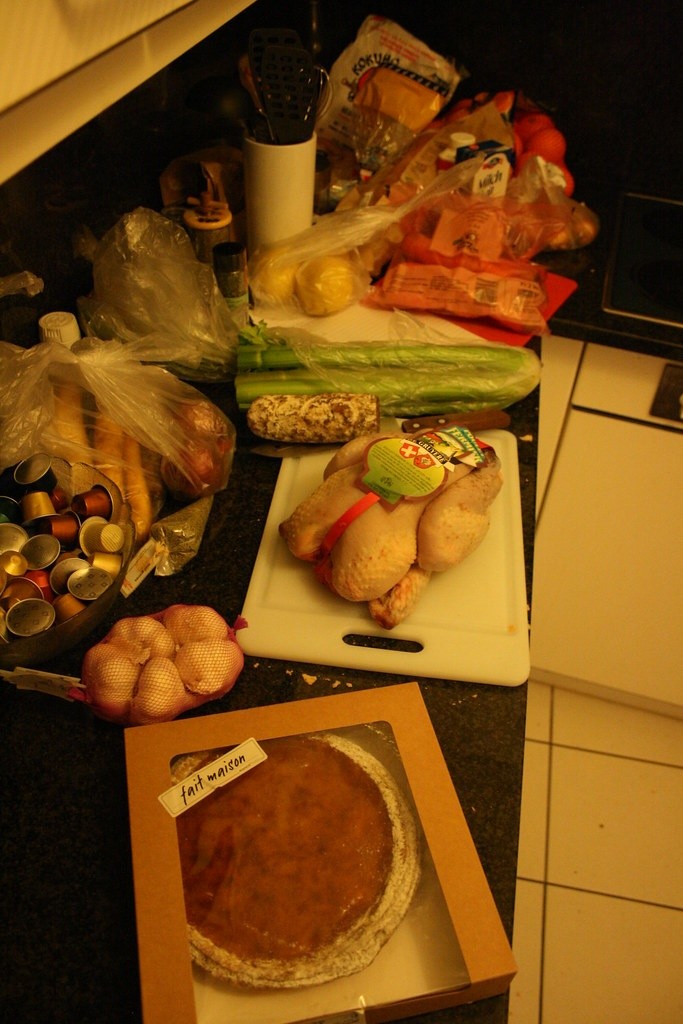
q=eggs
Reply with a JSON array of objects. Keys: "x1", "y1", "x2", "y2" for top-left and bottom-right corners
[{"x1": 512, "y1": 112, "x2": 575, "y2": 198}]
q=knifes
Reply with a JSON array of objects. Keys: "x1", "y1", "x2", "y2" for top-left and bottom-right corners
[{"x1": 249, "y1": 409, "x2": 509, "y2": 459}]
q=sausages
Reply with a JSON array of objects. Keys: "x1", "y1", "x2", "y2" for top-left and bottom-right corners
[{"x1": 247, "y1": 392, "x2": 381, "y2": 443}]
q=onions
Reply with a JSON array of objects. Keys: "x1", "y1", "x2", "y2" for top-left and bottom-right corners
[{"x1": 159, "y1": 401, "x2": 235, "y2": 497}]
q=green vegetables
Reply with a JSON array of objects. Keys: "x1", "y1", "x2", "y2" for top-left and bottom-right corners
[{"x1": 233, "y1": 318, "x2": 541, "y2": 417}]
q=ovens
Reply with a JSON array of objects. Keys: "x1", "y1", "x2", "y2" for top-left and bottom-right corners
[{"x1": 541, "y1": 128, "x2": 682, "y2": 364}]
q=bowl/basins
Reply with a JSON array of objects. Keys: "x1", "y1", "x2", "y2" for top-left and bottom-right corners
[{"x1": 0, "y1": 453, "x2": 137, "y2": 664}]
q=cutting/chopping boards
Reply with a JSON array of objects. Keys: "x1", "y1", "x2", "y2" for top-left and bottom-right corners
[{"x1": 232, "y1": 423, "x2": 533, "y2": 686}]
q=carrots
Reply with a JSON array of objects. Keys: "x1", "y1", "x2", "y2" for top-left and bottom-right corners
[
  {"x1": 398, "y1": 234, "x2": 508, "y2": 276},
  {"x1": 53, "y1": 385, "x2": 152, "y2": 537}
]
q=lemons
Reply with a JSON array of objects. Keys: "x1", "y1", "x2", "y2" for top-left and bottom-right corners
[{"x1": 247, "y1": 241, "x2": 355, "y2": 316}]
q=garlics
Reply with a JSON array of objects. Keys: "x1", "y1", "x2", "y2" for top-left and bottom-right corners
[{"x1": 83, "y1": 605, "x2": 243, "y2": 724}]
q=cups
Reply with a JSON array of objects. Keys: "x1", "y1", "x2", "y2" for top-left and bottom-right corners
[{"x1": 238, "y1": 132, "x2": 318, "y2": 257}]
